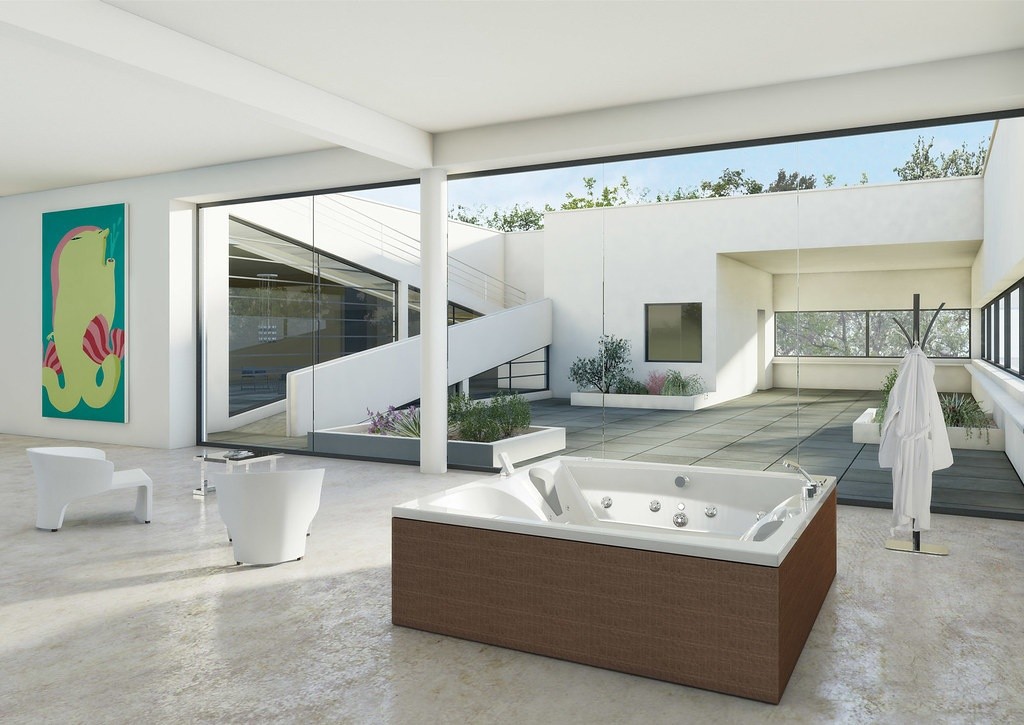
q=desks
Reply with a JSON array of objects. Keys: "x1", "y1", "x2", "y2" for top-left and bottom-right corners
[{"x1": 192, "y1": 449, "x2": 285, "y2": 496}]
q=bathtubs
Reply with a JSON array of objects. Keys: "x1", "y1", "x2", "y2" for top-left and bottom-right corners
[{"x1": 390, "y1": 454, "x2": 837, "y2": 705}]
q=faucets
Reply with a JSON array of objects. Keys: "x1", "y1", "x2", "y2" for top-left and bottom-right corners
[{"x1": 784, "y1": 459, "x2": 820, "y2": 495}]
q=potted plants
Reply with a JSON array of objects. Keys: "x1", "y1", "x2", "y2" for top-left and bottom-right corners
[
  {"x1": 853, "y1": 367, "x2": 1005, "y2": 451},
  {"x1": 568, "y1": 333, "x2": 714, "y2": 411},
  {"x1": 308, "y1": 388, "x2": 566, "y2": 467}
]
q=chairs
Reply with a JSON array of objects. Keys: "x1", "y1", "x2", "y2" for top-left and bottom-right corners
[
  {"x1": 26, "y1": 447, "x2": 153, "y2": 531},
  {"x1": 241, "y1": 367, "x2": 269, "y2": 389},
  {"x1": 217, "y1": 468, "x2": 326, "y2": 566}
]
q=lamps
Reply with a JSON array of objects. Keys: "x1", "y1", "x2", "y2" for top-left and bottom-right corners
[{"x1": 257, "y1": 273, "x2": 278, "y2": 343}]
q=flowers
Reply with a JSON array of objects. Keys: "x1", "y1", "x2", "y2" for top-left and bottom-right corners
[{"x1": 366, "y1": 404, "x2": 420, "y2": 437}]
